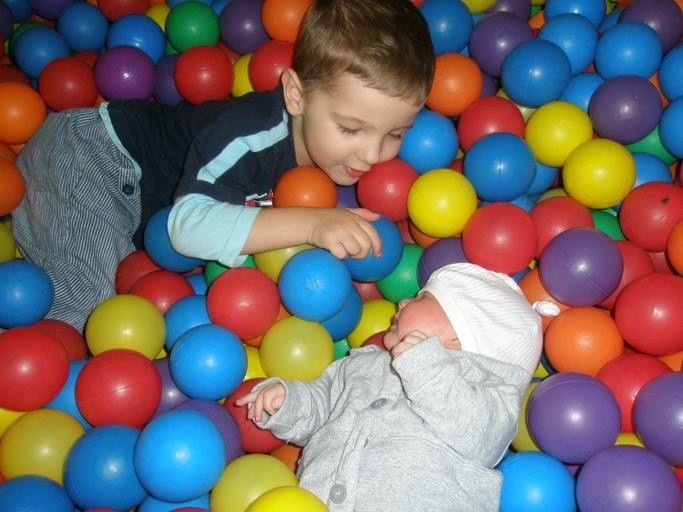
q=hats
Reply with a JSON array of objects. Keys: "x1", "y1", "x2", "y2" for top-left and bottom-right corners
[{"x1": 414, "y1": 260, "x2": 562, "y2": 377}]
